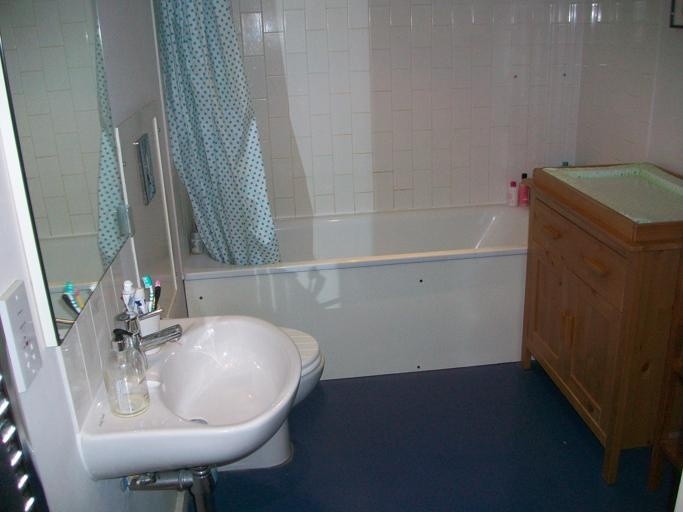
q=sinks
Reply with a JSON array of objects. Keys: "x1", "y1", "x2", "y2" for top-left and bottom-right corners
[
  {"x1": 82, "y1": 315, "x2": 302, "y2": 479},
  {"x1": 50, "y1": 285, "x2": 98, "y2": 346}
]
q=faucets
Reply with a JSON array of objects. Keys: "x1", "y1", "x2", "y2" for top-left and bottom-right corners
[{"x1": 116, "y1": 313, "x2": 182, "y2": 369}]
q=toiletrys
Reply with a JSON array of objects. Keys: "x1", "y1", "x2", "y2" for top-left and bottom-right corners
[
  {"x1": 104, "y1": 329, "x2": 148, "y2": 417},
  {"x1": 517, "y1": 173, "x2": 530, "y2": 206},
  {"x1": 504, "y1": 179, "x2": 517, "y2": 206},
  {"x1": 122, "y1": 280, "x2": 136, "y2": 313}
]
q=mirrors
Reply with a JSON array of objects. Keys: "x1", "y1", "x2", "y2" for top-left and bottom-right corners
[{"x1": 0, "y1": 0, "x2": 129, "y2": 345}]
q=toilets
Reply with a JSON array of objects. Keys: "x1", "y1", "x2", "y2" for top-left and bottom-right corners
[{"x1": 216, "y1": 328, "x2": 324, "y2": 472}]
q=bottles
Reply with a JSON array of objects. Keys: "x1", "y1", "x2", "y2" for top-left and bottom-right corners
[{"x1": 509, "y1": 172, "x2": 529, "y2": 207}]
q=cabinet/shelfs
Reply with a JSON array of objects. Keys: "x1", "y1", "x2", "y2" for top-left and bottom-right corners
[{"x1": 521, "y1": 187, "x2": 683, "y2": 484}]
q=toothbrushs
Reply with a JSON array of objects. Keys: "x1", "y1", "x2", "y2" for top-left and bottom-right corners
[
  {"x1": 62, "y1": 282, "x2": 84, "y2": 315},
  {"x1": 142, "y1": 276, "x2": 154, "y2": 312},
  {"x1": 154, "y1": 280, "x2": 161, "y2": 308}
]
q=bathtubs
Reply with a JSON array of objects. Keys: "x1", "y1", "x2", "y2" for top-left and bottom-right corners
[
  {"x1": 181, "y1": 204, "x2": 532, "y2": 379},
  {"x1": 34, "y1": 235, "x2": 104, "y2": 283}
]
